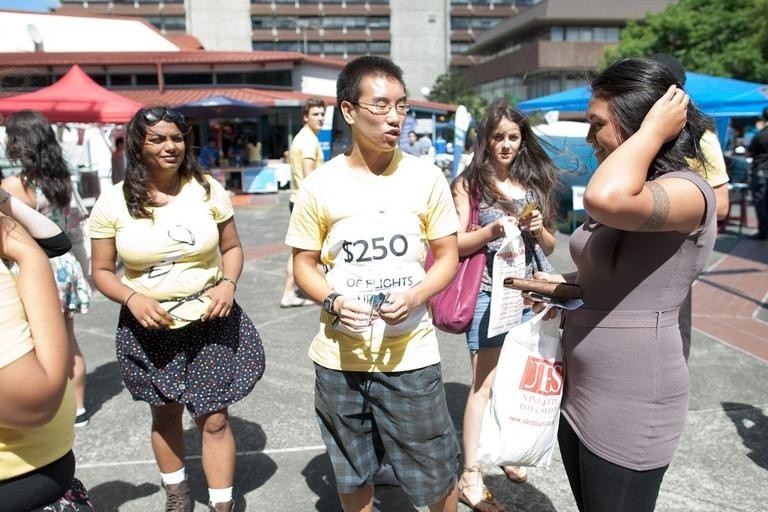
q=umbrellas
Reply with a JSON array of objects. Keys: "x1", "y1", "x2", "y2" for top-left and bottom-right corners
[{"x1": 165, "y1": 95, "x2": 272, "y2": 183}]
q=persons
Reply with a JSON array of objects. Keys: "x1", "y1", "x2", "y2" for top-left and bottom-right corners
[
  {"x1": 522, "y1": 57, "x2": 717, "y2": 511},
  {"x1": 403, "y1": 130, "x2": 457, "y2": 175},
  {"x1": 199, "y1": 131, "x2": 263, "y2": 190},
  {"x1": 111, "y1": 138, "x2": 127, "y2": 183},
  {"x1": 1, "y1": 108, "x2": 94, "y2": 512},
  {"x1": 281, "y1": 55, "x2": 463, "y2": 512},
  {"x1": 279, "y1": 98, "x2": 327, "y2": 308},
  {"x1": 450, "y1": 100, "x2": 559, "y2": 511},
  {"x1": 87, "y1": 103, "x2": 266, "y2": 511},
  {"x1": 651, "y1": 51, "x2": 767, "y2": 366}
]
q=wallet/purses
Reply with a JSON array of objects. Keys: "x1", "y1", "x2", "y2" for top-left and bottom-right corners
[{"x1": 504, "y1": 277, "x2": 583, "y2": 300}]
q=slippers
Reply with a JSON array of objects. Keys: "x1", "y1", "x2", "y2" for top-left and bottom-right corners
[{"x1": 278, "y1": 298, "x2": 314, "y2": 308}]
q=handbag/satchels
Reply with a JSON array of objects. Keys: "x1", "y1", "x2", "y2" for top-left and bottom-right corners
[
  {"x1": 65, "y1": 224, "x2": 122, "y2": 280},
  {"x1": 425, "y1": 237, "x2": 487, "y2": 333}
]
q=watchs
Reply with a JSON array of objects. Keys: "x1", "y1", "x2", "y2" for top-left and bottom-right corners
[{"x1": 323, "y1": 291, "x2": 343, "y2": 328}]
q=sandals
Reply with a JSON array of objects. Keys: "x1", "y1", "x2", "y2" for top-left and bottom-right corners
[
  {"x1": 502, "y1": 466, "x2": 528, "y2": 483},
  {"x1": 458, "y1": 470, "x2": 503, "y2": 512}
]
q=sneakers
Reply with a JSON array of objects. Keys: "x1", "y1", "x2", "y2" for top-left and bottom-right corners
[
  {"x1": 74, "y1": 413, "x2": 89, "y2": 428},
  {"x1": 160, "y1": 473, "x2": 192, "y2": 511},
  {"x1": 207, "y1": 500, "x2": 234, "y2": 512}
]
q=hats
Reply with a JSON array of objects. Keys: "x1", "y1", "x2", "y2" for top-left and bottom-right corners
[{"x1": 648, "y1": 53, "x2": 686, "y2": 85}]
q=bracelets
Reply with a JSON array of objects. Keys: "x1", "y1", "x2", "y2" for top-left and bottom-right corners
[
  {"x1": 222, "y1": 276, "x2": 237, "y2": 292},
  {"x1": 124, "y1": 290, "x2": 136, "y2": 307}
]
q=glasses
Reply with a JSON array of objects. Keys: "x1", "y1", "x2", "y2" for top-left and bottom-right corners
[
  {"x1": 143, "y1": 108, "x2": 185, "y2": 126},
  {"x1": 351, "y1": 101, "x2": 410, "y2": 115}
]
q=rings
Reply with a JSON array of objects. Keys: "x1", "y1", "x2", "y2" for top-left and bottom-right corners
[{"x1": 145, "y1": 317, "x2": 152, "y2": 324}]
